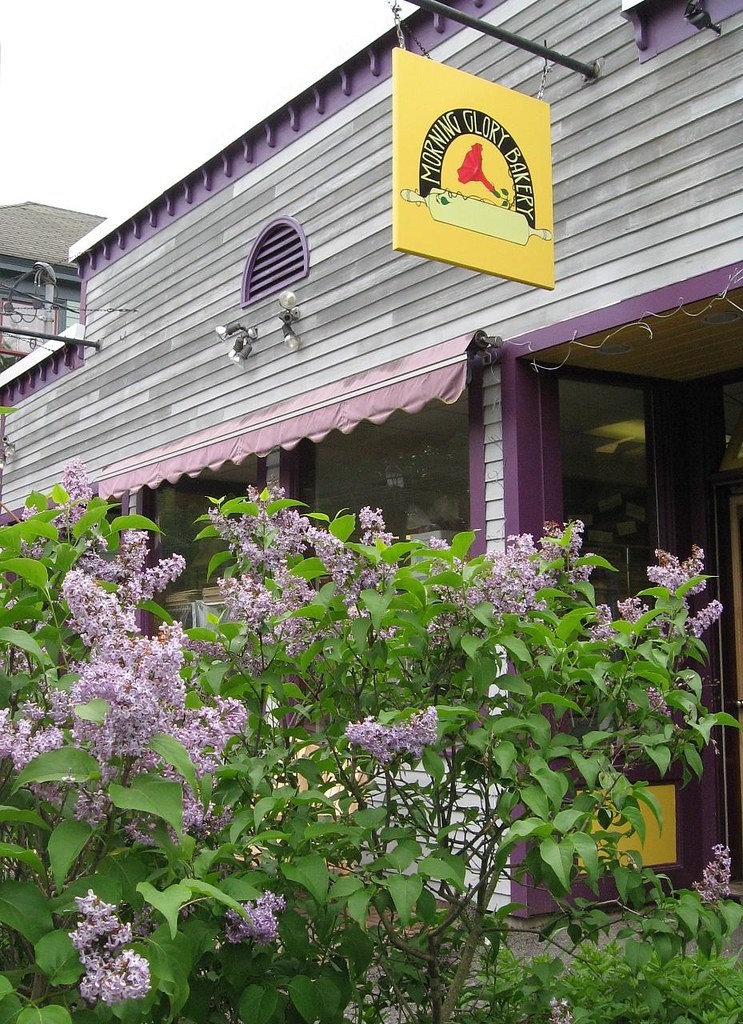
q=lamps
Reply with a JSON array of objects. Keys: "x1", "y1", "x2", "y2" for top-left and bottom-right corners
[
  {"x1": 276, "y1": 291, "x2": 302, "y2": 351},
  {"x1": 0, "y1": 436, "x2": 16, "y2": 469},
  {"x1": 215, "y1": 324, "x2": 258, "y2": 372}
]
